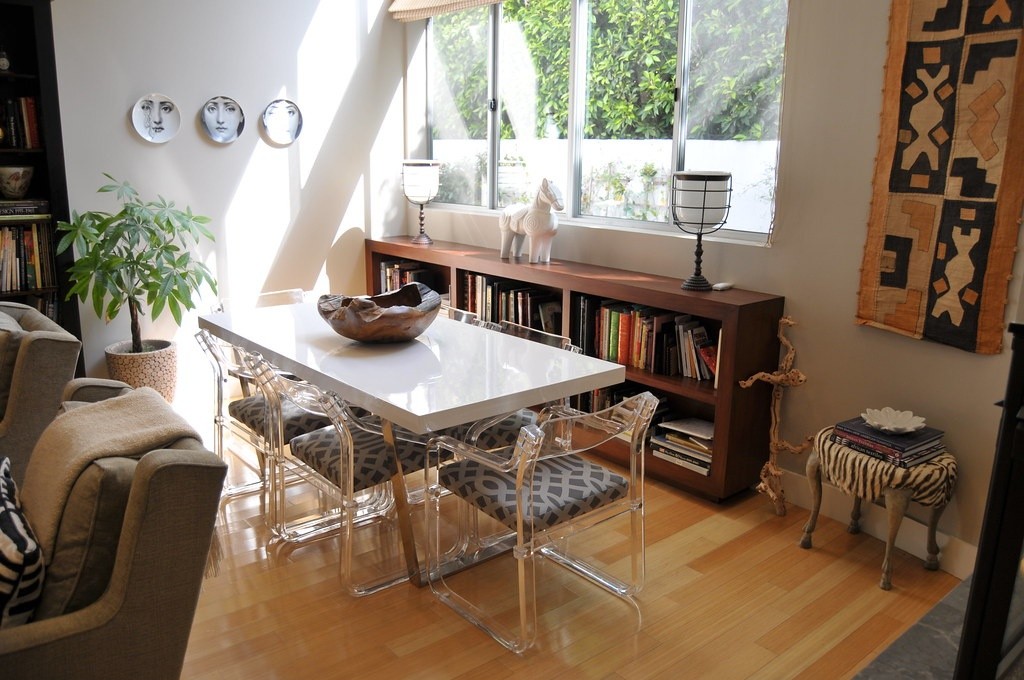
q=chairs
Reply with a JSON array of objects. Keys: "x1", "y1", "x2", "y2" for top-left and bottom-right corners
[
  {"x1": 220, "y1": 289, "x2": 306, "y2": 397},
  {"x1": 426, "y1": 392, "x2": 660, "y2": 654},
  {"x1": 438, "y1": 303, "x2": 582, "y2": 549},
  {"x1": 244, "y1": 348, "x2": 464, "y2": 597},
  {"x1": 194, "y1": 327, "x2": 317, "y2": 519}
]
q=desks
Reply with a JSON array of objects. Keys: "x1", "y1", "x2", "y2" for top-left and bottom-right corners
[{"x1": 199, "y1": 305, "x2": 626, "y2": 589}]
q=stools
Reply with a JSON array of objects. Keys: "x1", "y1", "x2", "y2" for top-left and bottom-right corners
[{"x1": 800, "y1": 425, "x2": 958, "y2": 591}]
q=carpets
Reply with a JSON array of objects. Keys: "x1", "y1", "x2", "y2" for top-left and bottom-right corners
[{"x1": 851, "y1": 568, "x2": 1024, "y2": 680}]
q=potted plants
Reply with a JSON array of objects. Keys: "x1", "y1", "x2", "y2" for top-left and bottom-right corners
[
  {"x1": 475, "y1": 150, "x2": 527, "y2": 191},
  {"x1": 55, "y1": 172, "x2": 218, "y2": 403}
]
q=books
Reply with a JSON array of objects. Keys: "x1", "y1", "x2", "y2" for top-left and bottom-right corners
[
  {"x1": 0, "y1": 199, "x2": 63, "y2": 325},
  {"x1": 462, "y1": 271, "x2": 562, "y2": 346},
  {"x1": 0, "y1": 96, "x2": 39, "y2": 150},
  {"x1": 570, "y1": 292, "x2": 723, "y2": 477},
  {"x1": 829, "y1": 416, "x2": 946, "y2": 468},
  {"x1": 380, "y1": 260, "x2": 451, "y2": 318}
]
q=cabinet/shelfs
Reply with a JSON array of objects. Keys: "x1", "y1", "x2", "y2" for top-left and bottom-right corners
[
  {"x1": 0, "y1": 0, "x2": 86, "y2": 377},
  {"x1": 589, "y1": 163, "x2": 667, "y2": 223},
  {"x1": 364, "y1": 236, "x2": 786, "y2": 506}
]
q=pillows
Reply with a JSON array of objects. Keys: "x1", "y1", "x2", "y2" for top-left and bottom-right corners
[{"x1": 0, "y1": 456, "x2": 46, "y2": 629}]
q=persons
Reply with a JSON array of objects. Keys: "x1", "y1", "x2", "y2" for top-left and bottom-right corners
[
  {"x1": 262, "y1": 99, "x2": 303, "y2": 144},
  {"x1": 201, "y1": 96, "x2": 244, "y2": 143},
  {"x1": 140, "y1": 94, "x2": 180, "y2": 142}
]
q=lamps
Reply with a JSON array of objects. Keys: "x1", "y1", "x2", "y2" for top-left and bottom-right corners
[
  {"x1": 670, "y1": 170, "x2": 733, "y2": 291},
  {"x1": 401, "y1": 159, "x2": 440, "y2": 245}
]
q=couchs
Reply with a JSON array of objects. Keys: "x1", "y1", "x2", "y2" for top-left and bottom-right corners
[{"x1": 0, "y1": 300, "x2": 228, "y2": 680}]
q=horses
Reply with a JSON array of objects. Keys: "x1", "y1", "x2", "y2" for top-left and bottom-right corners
[{"x1": 499, "y1": 178, "x2": 564, "y2": 264}]
